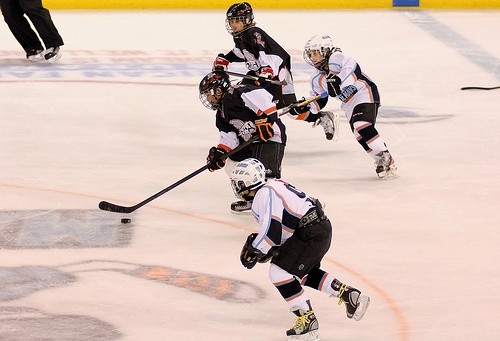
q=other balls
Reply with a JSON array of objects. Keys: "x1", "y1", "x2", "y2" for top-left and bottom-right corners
[{"x1": 121, "y1": 218, "x2": 132, "y2": 223}]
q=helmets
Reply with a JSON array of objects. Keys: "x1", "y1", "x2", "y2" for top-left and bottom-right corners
[
  {"x1": 303, "y1": 35, "x2": 336, "y2": 71},
  {"x1": 225, "y1": 2, "x2": 256, "y2": 38},
  {"x1": 230, "y1": 157, "x2": 266, "y2": 203},
  {"x1": 199, "y1": 72, "x2": 231, "y2": 110}
]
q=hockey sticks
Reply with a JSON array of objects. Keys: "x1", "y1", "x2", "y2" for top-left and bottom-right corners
[
  {"x1": 98, "y1": 135, "x2": 260, "y2": 214},
  {"x1": 267, "y1": 94, "x2": 321, "y2": 117},
  {"x1": 224, "y1": 68, "x2": 292, "y2": 86},
  {"x1": 461, "y1": 86, "x2": 500, "y2": 90}
]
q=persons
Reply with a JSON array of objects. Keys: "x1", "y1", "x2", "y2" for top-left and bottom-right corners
[
  {"x1": 288, "y1": 34, "x2": 400, "y2": 181},
  {"x1": 231, "y1": 158, "x2": 370, "y2": 341},
  {"x1": 212, "y1": 2, "x2": 339, "y2": 142},
  {"x1": 197, "y1": 70, "x2": 287, "y2": 214},
  {"x1": 0, "y1": 0, "x2": 65, "y2": 64}
]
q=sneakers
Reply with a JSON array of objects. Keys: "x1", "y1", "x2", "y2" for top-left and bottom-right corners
[
  {"x1": 27, "y1": 45, "x2": 46, "y2": 62},
  {"x1": 287, "y1": 300, "x2": 319, "y2": 341},
  {"x1": 231, "y1": 199, "x2": 252, "y2": 215},
  {"x1": 373, "y1": 143, "x2": 397, "y2": 180},
  {"x1": 45, "y1": 45, "x2": 62, "y2": 64},
  {"x1": 313, "y1": 111, "x2": 338, "y2": 140},
  {"x1": 331, "y1": 278, "x2": 370, "y2": 321}
]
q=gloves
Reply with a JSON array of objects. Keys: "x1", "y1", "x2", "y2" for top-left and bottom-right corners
[
  {"x1": 207, "y1": 147, "x2": 229, "y2": 172},
  {"x1": 287, "y1": 97, "x2": 311, "y2": 115},
  {"x1": 326, "y1": 73, "x2": 341, "y2": 98},
  {"x1": 240, "y1": 233, "x2": 263, "y2": 269},
  {"x1": 257, "y1": 246, "x2": 281, "y2": 264},
  {"x1": 212, "y1": 54, "x2": 229, "y2": 73},
  {"x1": 255, "y1": 117, "x2": 274, "y2": 143},
  {"x1": 254, "y1": 65, "x2": 274, "y2": 88}
]
